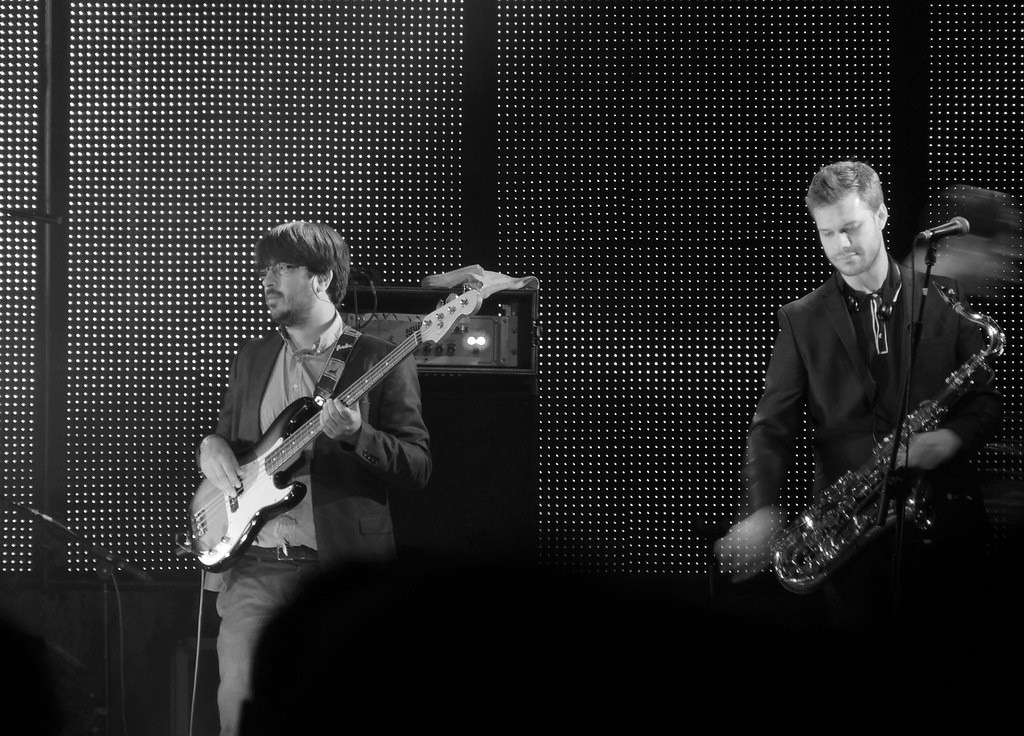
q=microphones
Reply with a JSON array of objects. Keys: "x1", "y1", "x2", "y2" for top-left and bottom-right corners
[{"x1": 917, "y1": 217, "x2": 970, "y2": 241}]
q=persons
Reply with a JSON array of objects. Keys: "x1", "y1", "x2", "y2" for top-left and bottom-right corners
[
  {"x1": 714, "y1": 161, "x2": 1004, "y2": 575},
  {"x1": 196, "y1": 221, "x2": 435, "y2": 736}
]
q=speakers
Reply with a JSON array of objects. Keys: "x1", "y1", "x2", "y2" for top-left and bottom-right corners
[{"x1": 383, "y1": 373, "x2": 541, "y2": 562}]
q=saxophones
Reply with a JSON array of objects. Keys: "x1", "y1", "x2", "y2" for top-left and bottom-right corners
[{"x1": 769, "y1": 279, "x2": 1006, "y2": 596}]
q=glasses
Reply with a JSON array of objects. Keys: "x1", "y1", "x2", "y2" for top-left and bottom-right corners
[{"x1": 258, "y1": 264, "x2": 306, "y2": 281}]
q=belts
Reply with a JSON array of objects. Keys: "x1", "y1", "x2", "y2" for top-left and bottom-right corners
[{"x1": 245, "y1": 545, "x2": 318, "y2": 560}]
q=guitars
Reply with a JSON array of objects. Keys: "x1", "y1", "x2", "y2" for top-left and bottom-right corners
[{"x1": 176, "y1": 285, "x2": 484, "y2": 572}]
